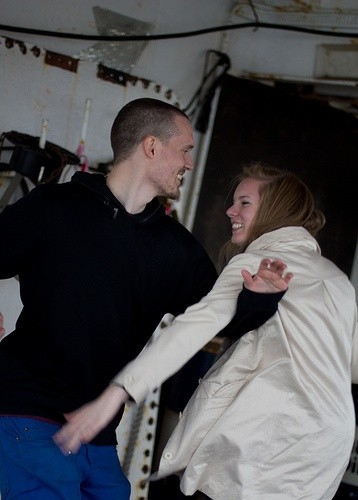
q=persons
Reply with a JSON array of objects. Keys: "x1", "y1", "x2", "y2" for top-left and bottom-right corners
[
  {"x1": 1, "y1": 98, "x2": 293, "y2": 500},
  {"x1": 51, "y1": 162, "x2": 358, "y2": 500}
]
